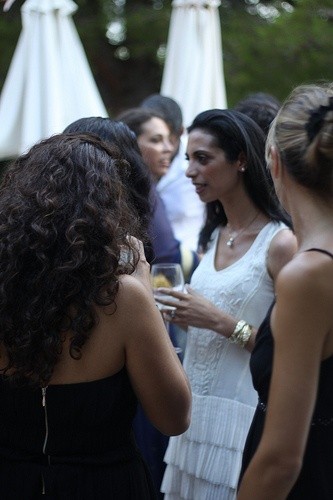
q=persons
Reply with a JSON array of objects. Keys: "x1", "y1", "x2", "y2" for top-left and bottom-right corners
[
  {"x1": 148, "y1": 107, "x2": 295, "y2": 499},
  {"x1": 66, "y1": 91, "x2": 280, "y2": 500},
  {"x1": 236, "y1": 80, "x2": 333, "y2": 500},
  {"x1": 3, "y1": 131, "x2": 193, "y2": 499}
]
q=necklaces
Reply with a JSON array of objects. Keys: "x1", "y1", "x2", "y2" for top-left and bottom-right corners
[{"x1": 226, "y1": 210, "x2": 260, "y2": 248}]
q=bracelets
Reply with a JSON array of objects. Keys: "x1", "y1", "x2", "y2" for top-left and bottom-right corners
[{"x1": 230, "y1": 319, "x2": 253, "y2": 348}]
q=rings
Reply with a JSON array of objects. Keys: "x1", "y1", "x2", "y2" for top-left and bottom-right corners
[{"x1": 170, "y1": 310, "x2": 175, "y2": 317}]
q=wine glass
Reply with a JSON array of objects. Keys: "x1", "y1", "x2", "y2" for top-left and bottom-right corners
[{"x1": 148, "y1": 263, "x2": 185, "y2": 353}]
